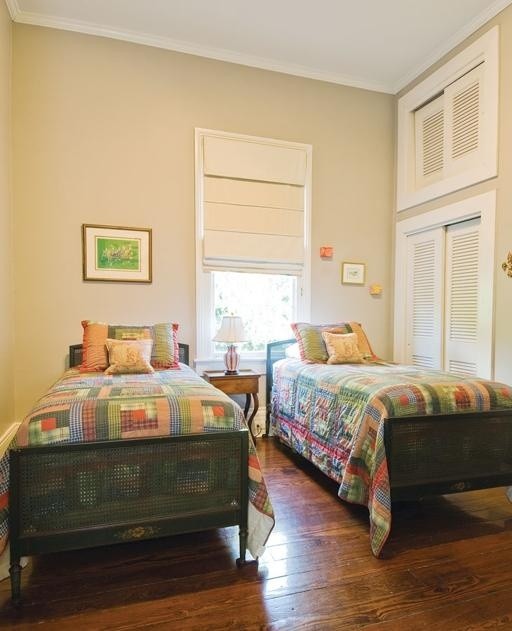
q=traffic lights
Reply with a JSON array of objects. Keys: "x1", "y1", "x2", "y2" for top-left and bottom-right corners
[
  {"x1": 320, "y1": 331, "x2": 369, "y2": 365},
  {"x1": 104, "y1": 337, "x2": 155, "y2": 375},
  {"x1": 289, "y1": 321, "x2": 378, "y2": 365},
  {"x1": 285, "y1": 342, "x2": 301, "y2": 360},
  {"x1": 79, "y1": 320, "x2": 181, "y2": 372}
]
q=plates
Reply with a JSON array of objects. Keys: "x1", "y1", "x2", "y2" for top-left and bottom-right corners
[
  {"x1": 389, "y1": 191, "x2": 496, "y2": 382},
  {"x1": 396, "y1": 23, "x2": 499, "y2": 215}
]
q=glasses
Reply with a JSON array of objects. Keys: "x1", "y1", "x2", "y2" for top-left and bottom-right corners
[{"x1": 211, "y1": 315, "x2": 249, "y2": 375}]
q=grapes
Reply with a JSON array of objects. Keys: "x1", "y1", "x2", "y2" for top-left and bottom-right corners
[{"x1": 203, "y1": 368, "x2": 261, "y2": 447}]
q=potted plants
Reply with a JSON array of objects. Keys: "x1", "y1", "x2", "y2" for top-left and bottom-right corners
[
  {"x1": 341, "y1": 262, "x2": 366, "y2": 285},
  {"x1": 81, "y1": 223, "x2": 153, "y2": 284}
]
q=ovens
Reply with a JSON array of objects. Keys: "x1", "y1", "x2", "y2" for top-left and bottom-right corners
[
  {"x1": 262, "y1": 337, "x2": 511, "y2": 558},
  {"x1": 1, "y1": 340, "x2": 275, "y2": 600}
]
